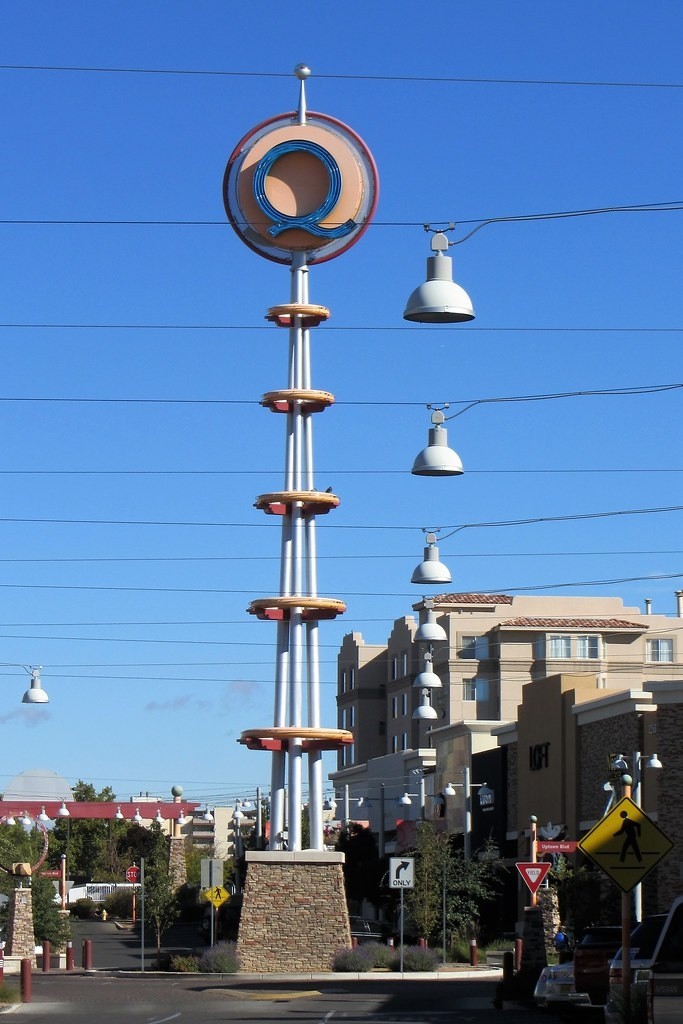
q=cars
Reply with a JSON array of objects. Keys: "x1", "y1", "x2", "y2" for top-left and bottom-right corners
[{"x1": 528, "y1": 896, "x2": 683, "y2": 1024}]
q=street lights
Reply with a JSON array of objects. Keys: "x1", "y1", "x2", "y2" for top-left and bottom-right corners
[
  {"x1": 232, "y1": 798, "x2": 245, "y2": 897},
  {"x1": 245, "y1": 786, "x2": 270, "y2": 851},
  {"x1": 325, "y1": 783, "x2": 365, "y2": 850},
  {"x1": 607, "y1": 751, "x2": 662, "y2": 924},
  {"x1": 444, "y1": 766, "x2": 489, "y2": 944},
  {"x1": 400, "y1": 778, "x2": 446, "y2": 947}
]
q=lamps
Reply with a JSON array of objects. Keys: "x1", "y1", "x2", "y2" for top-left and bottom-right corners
[{"x1": 322, "y1": 780, "x2": 491, "y2": 810}]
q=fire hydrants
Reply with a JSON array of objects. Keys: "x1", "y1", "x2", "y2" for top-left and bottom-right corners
[{"x1": 102, "y1": 909, "x2": 109, "y2": 922}]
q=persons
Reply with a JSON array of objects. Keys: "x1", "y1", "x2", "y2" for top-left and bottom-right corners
[{"x1": 554, "y1": 926, "x2": 572, "y2": 951}]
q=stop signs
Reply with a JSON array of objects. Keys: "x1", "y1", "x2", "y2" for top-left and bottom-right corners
[{"x1": 126, "y1": 866, "x2": 141, "y2": 883}]
